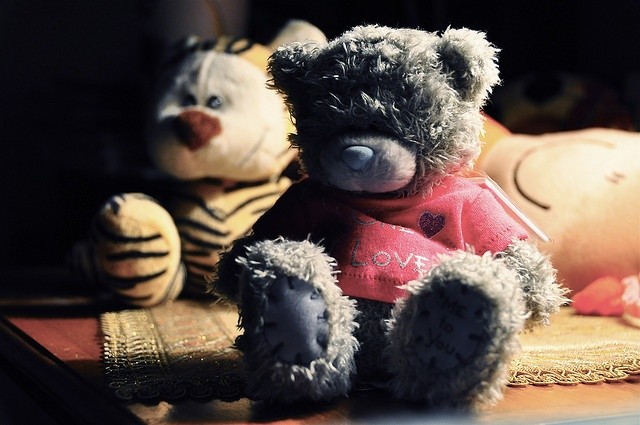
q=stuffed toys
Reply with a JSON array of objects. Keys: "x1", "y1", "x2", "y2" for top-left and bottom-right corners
[
  {"x1": 202, "y1": 24, "x2": 572, "y2": 409},
  {"x1": 98, "y1": 17, "x2": 330, "y2": 304},
  {"x1": 473, "y1": 109, "x2": 640, "y2": 296}
]
